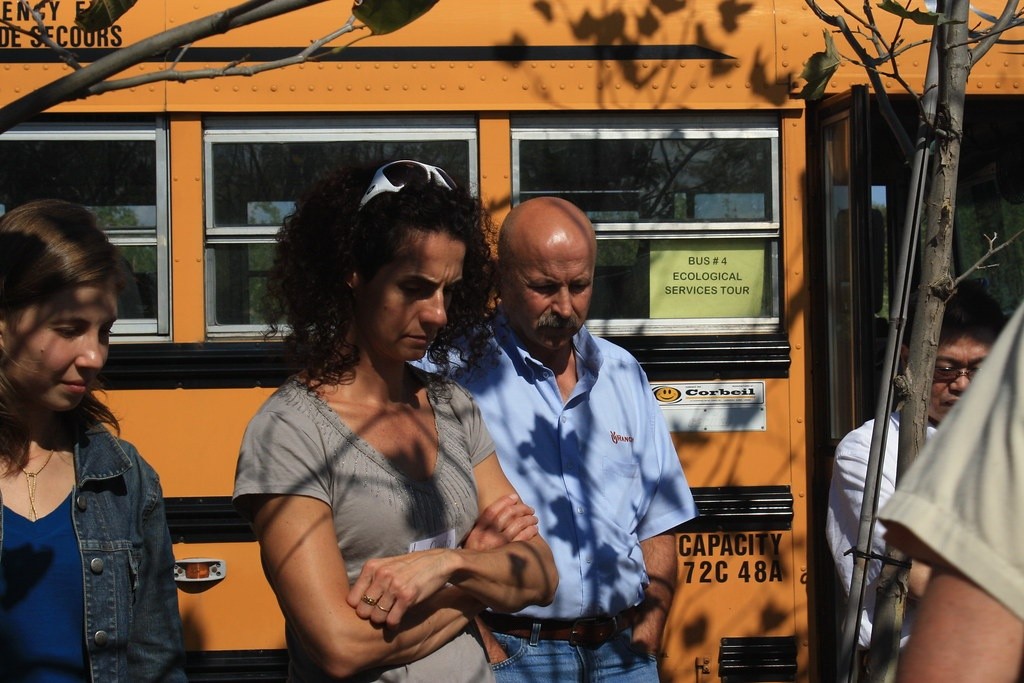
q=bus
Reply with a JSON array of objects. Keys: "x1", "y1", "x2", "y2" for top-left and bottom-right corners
[{"x1": 0, "y1": 0, "x2": 1024, "y2": 683}]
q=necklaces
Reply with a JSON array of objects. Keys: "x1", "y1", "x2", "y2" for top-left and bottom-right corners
[{"x1": 0, "y1": 424, "x2": 62, "y2": 523}]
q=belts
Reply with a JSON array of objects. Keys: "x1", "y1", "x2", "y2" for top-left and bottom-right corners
[{"x1": 470, "y1": 608, "x2": 643, "y2": 663}]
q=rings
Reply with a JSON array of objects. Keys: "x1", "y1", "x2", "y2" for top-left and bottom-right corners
[
  {"x1": 376, "y1": 602, "x2": 390, "y2": 613},
  {"x1": 361, "y1": 594, "x2": 376, "y2": 606}
]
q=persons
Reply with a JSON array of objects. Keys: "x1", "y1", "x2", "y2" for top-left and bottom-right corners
[
  {"x1": 232, "y1": 159, "x2": 560, "y2": 683},
  {"x1": 0, "y1": 198, "x2": 194, "y2": 683},
  {"x1": 823, "y1": 278, "x2": 1024, "y2": 683},
  {"x1": 413, "y1": 194, "x2": 700, "y2": 683}
]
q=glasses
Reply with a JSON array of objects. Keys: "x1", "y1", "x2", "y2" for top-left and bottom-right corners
[
  {"x1": 356, "y1": 161, "x2": 459, "y2": 213},
  {"x1": 933, "y1": 366, "x2": 981, "y2": 383}
]
